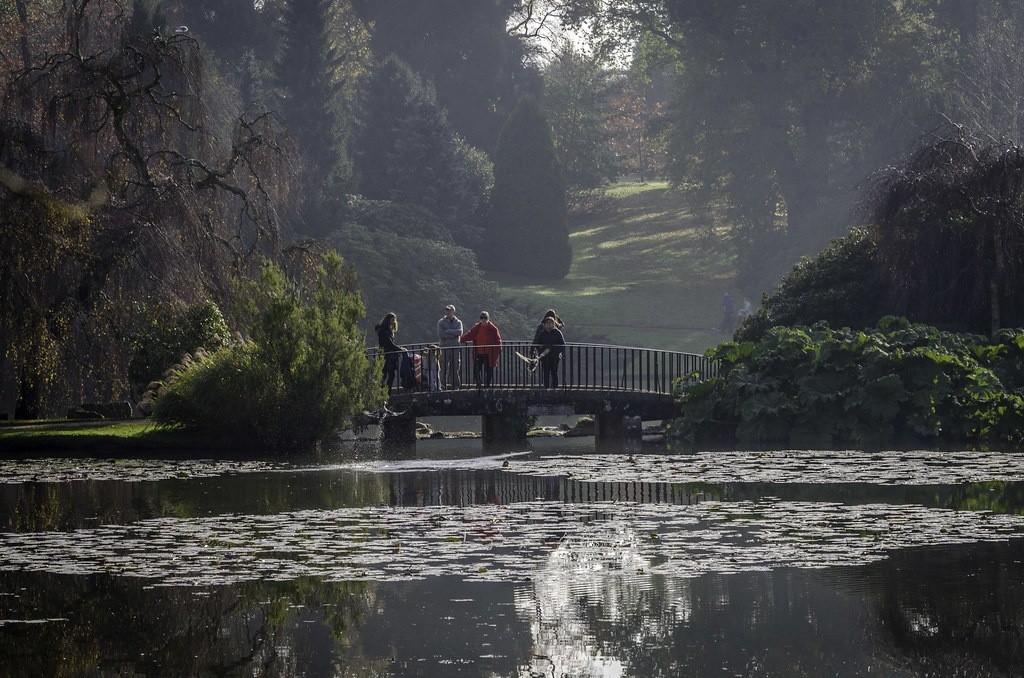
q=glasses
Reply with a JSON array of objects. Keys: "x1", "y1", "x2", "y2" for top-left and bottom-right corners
[{"x1": 480, "y1": 317, "x2": 488, "y2": 320}]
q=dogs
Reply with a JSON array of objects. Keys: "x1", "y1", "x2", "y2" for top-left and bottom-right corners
[{"x1": 420, "y1": 345, "x2": 443, "y2": 392}]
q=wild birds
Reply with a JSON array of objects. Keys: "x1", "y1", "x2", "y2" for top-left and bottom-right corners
[{"x1": 515, "y1": 348, "x2": 550, "y2": 372}]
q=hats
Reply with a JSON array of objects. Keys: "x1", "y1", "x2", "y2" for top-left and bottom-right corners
[
  {"x1": 545, "y1": 317, "x2": 554, "y2": 322},
  {"x1": 445, "y1": 305, "x2": 455, "y2": 311}
]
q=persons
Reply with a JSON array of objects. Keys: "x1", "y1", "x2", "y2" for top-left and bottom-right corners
[
  {"x1": 437, "y1": 306, "x2": 462, "y2": 390},
  {"x1": 720, "y1": 292, "x2": 754, "y2": 334},
  {"x1": 528, "y1": 309, "x2": 565, "y2": 389},
  {"x1": 377, "y1": 312, "x2": 410, "y2": 390},
  {"x1": 461, "y1": 312, "x2": 502, "y2": 386}
]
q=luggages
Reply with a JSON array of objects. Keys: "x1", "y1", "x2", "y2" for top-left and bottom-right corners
[{"x1": 400, "y1": 348, "x2": 423, "y2": 388}]
